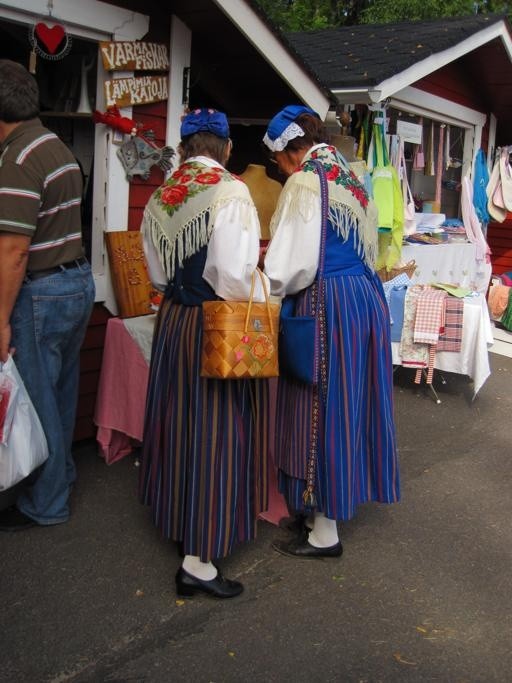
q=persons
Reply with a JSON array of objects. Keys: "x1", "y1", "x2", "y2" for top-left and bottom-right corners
[
  {"x1": 260, "y1": 103, "x2": 402, "y2": 563},
  {"x1": 137, "y1": 104, "x2": 274, "y2": 602},
  {"x1": 0, "y1": 58, "x2": 98, "y2": 532}
]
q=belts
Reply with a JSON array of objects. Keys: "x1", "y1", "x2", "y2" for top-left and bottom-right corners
[{"x1": 21, "y1": 256, "x2": 88, "y2": 285}]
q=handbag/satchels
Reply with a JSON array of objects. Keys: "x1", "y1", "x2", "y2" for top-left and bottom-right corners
[
  {"x1": 274, "y1": 291, "x2": 331, "y2": 391},
  {"x1": 198, "y1": 264, "x2": 284, "y2": 384}
]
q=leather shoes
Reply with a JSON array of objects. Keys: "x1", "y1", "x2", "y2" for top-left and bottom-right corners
[
  {"x1": 0, "y1": 502, "x2": 41, "y2": 533},
  {"x1": 174, "y1": 565, "x2": 244, "y2": 601},
  {"x1": 278, "y1": 511, "x2": 315, "y2": 535},
  {"x1": 269, "y1": 531, "x2": 345, "y2": 562}
]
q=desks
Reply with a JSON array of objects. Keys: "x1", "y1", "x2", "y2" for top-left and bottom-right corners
[
  {"x1": 379, "y1": 287, "x2": 484, "y2": 404},
  {"x1": 105, "y1": 313, "x2": 158, "y2": 465}
]
